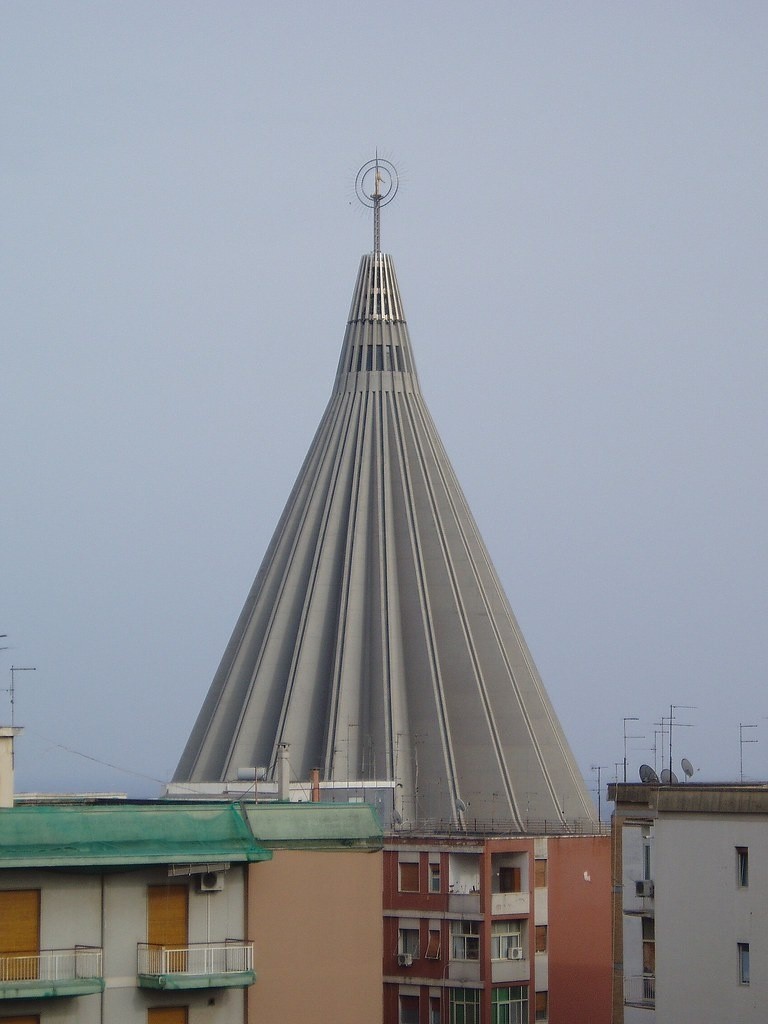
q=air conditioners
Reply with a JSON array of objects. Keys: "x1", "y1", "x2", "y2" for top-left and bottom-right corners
[
  {"x1": 635, "y1": 880, "x2": 653, "y2": 897},
  {"x1": 200, "y1": 872, "x2": 224, "y2": 890},
  {"x1": 508, "y1": 947, "x2": 522, "y2": 959},
  {"x1": 397, "y1": 954, "x2": 412, "y2": 964}
]
